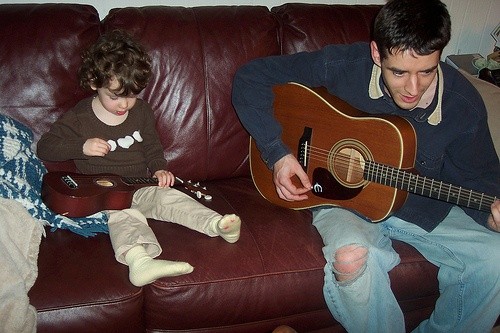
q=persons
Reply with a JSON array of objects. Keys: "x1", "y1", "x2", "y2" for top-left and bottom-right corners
[
  {"x1": 37, "y1": 30, "x2": 241, "y2": 287},
  {"x1": 231, "y1": 0, "x2": 500, "y2": 333}
]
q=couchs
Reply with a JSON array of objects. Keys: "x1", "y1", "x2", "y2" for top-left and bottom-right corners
[{"x1": 0, "y1": 2, "x2": 500, "y2": 333}]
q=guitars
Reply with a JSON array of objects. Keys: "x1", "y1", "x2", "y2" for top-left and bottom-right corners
[
  {"x1": 41, "y1": 171, "x2": 212, "y2": 218},
  {"x1": 249, "y1": 82, "x2": 500, "y2": 223}
]
